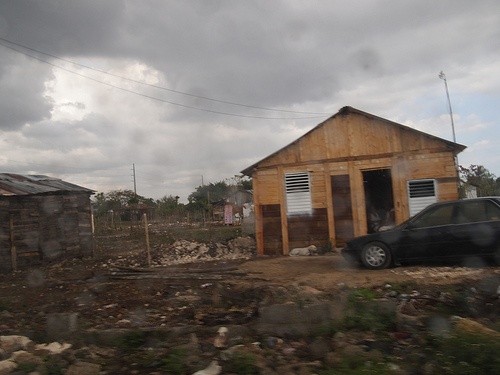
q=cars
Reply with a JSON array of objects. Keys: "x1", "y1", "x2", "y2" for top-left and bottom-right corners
[{"x1": 341, "y1": 196, "x2": 500, "y2": 270}]
